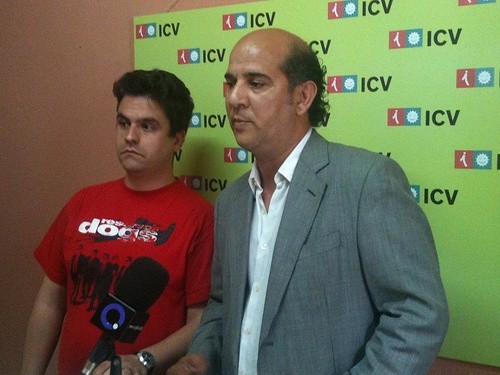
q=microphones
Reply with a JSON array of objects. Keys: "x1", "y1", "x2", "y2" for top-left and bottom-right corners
[{"x1": 81, "y1": 257, "x2": 169, "y2": 375}]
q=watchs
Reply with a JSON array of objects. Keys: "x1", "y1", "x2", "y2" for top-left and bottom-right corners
[{"x1": 133, "y1": 350, "x2": 157, "y2": 375}]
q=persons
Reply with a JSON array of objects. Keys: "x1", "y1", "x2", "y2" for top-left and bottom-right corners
[
  {"x1": 16, "y1": 68, "x2": 214, "y2": 375},
  {"x1": 166, "y1": 27, "x2": 450, "y2": 375}
]
